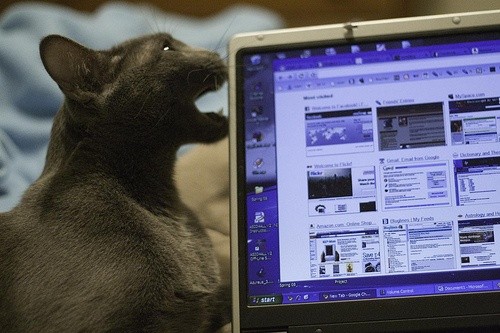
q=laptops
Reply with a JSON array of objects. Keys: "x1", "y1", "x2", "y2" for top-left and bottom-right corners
[{"x1": 226, "y1": 8, "x2": 499, "y2": 333}]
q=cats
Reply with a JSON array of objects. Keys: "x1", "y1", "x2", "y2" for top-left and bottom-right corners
[{"x1": 1, "y1": 14, "x2": 233, "y2": 332}]
